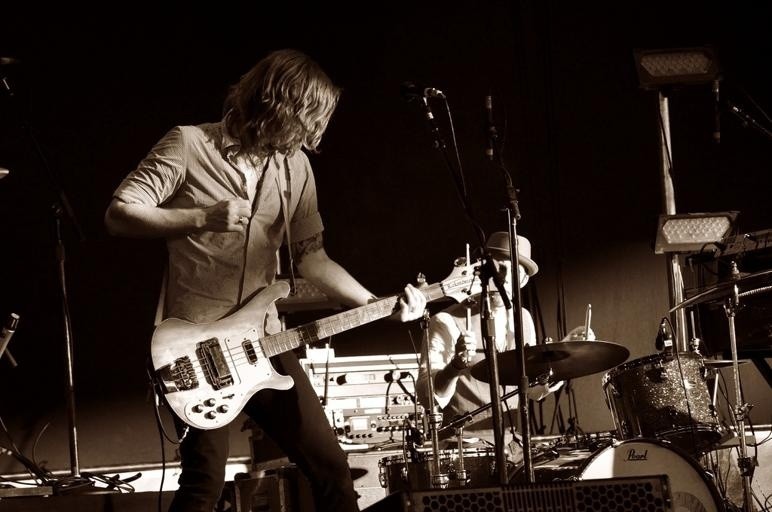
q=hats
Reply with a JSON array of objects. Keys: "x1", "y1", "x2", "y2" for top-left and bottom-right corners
[{"x1": 481, "y1": 231, "x2": 539, "y2": 277}]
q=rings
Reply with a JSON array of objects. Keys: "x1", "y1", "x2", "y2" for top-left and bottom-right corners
[{"x1": 238, "y1": 215, "x2": 244, "y2": 225}]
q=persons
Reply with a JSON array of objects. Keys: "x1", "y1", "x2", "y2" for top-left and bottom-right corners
[
  {"x1": 415, "y1": 230, "x2": 569, "y2": 449},
  {"x1": 105, "y1": 47, "x2": 428, "y2": 512}
]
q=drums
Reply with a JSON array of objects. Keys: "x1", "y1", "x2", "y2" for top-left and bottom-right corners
[
  {"x1": 508, "y1": 434, "x2": 725, "y2": 512},
  {"x1": 602, "y1": 351, "x2": 723, "y2": 457},
  {"x1": 377, "y1": 447, "x2": 500, "y2": 511}
]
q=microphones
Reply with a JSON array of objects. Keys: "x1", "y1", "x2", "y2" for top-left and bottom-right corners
[
  {"x1": 654, "y1": 322, "x2": 669, "y2": 350},
  {"x1": 0, "y1": 312, "x2": 20, "y2": 357},
  {"x1": 485, "y1": 95, "x2": 496, "y2": 161},
  {"x1": 399, "y1": 82, "x2": 441, "y2": 101},
  {"x1": 697, "y1": 241, "x2": 727, "y2": 292}
]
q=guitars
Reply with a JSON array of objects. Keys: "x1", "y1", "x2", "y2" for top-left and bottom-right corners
[{"x1": 150, "y1": 258, "x2": 501, "y2": 430}]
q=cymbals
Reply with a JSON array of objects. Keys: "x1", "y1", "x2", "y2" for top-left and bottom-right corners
[
  {"x1": 699, "y1": 360, "x2": 750, "y2": 369},
  {"x1": 469, "y1": 340, "x2": 629, "y2": 386}
]
